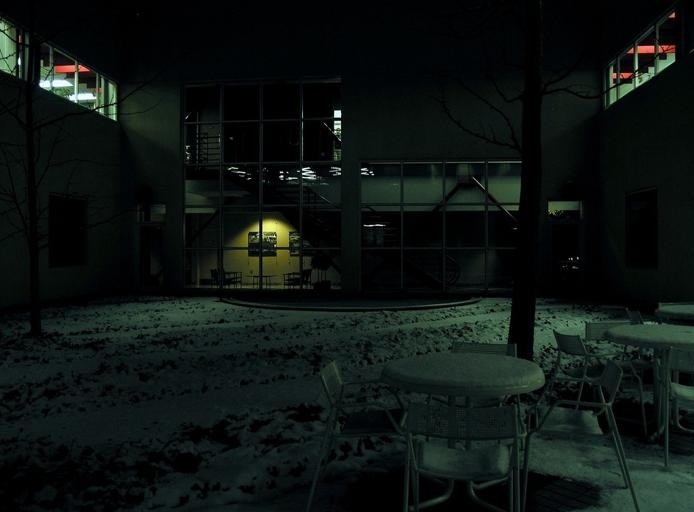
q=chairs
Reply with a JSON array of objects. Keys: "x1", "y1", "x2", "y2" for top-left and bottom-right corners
[
  {"x1": 540, "y1": 330, "x2": 648, "y2": 436},
  {"x1": 206, "y1": 265, "x2": 314, "y2": 291},
  {"x1": 304, "y1": 362, "x2": 418, "y2": 510},
  {"x1": 580, "y1": 318, "x2": 627, "y2": 341},
  {"x1": 428, "y1": 339, "x2": 520, "y2": 468},
  {"x1": 659, "y1": 343, "x2": 694, "y2": 471},
  {"x1": 525, "y1": 356, "x2": 642, "y2": 511}
]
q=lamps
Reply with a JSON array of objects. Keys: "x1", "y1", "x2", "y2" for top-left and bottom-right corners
[{"x1": 226, "y1": 160, "x2": 378, "y2": 186}]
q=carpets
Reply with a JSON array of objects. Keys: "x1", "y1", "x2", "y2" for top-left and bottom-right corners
[
  {"x1": 549, "y1": 383, "x2": 693, "y2": 448},
  {"x1": 620, "y1": 359, "x2": 694, "y2": 390}
]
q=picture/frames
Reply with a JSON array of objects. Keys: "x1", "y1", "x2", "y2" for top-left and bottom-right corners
[{"x1": 622, "y1": 185, "x2": 660, "y2": 268}]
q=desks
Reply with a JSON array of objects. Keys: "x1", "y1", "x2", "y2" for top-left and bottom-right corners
[
  {"x1": 651, "y1": 301, "x2": 693, "y2": 321},
  {"x1": 379, "y1": 350, "x2": 545, "y2": 510},
  {"x1": 604, "y1": 325, "x2": 694, "y2": 459}
]
